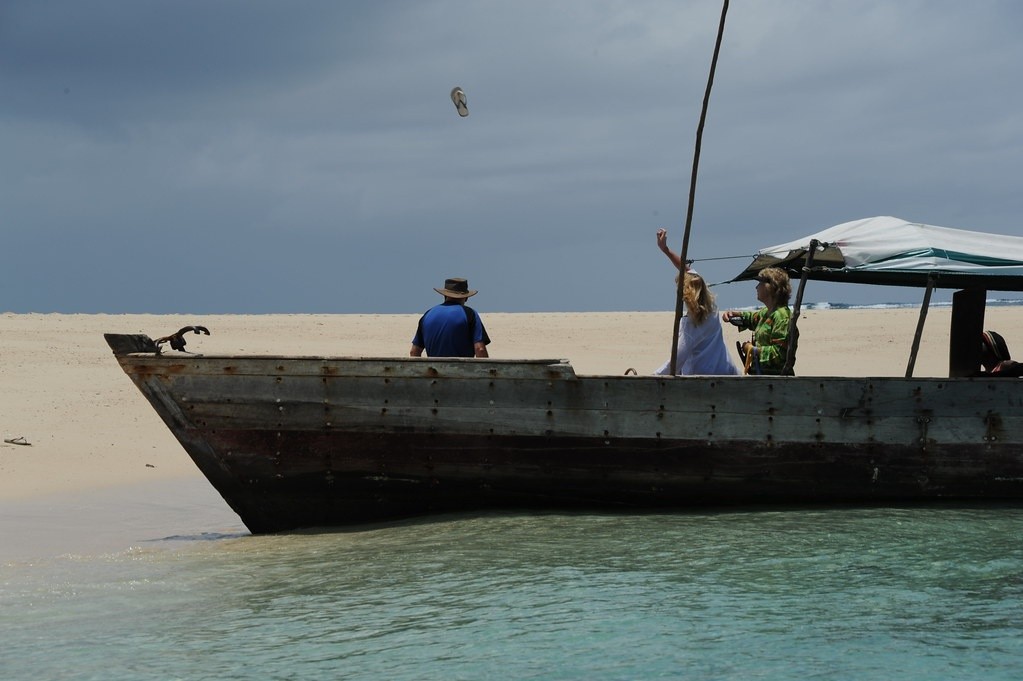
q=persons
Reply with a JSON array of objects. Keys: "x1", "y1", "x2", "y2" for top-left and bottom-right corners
[
  {"x1": 722, "y1": 268, "x2": 797, "y2": 376},
  {"x1": 658, "y1": 228, "x2": 739, "y2": 376},
  {"x1": 410, "y1": 277, "x2": 491, "y2": 359},
  {"x1": 983, "y1": 330, "x2": 1023, "y2": 372}
]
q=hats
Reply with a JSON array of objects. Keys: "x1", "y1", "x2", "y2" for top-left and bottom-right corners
[{"x1": 433, "y1": 278, "x2": 478, "y2": 298}]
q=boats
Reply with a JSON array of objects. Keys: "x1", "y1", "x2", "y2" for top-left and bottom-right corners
[{"x1": 103, "y1": 212, "x2": 1023, "y2": 535}]
